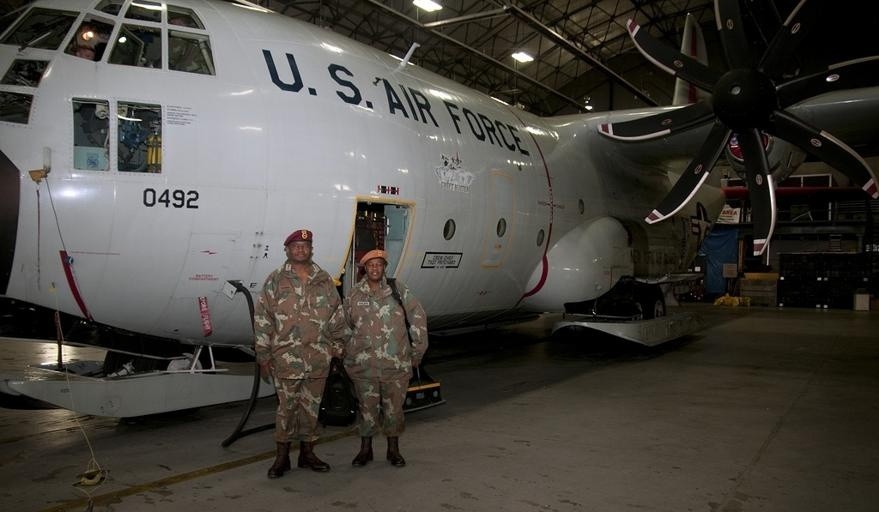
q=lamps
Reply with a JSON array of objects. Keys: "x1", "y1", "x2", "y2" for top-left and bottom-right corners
[{"x1": 583, "y1": 96, "x2": 593, "y2": 110}]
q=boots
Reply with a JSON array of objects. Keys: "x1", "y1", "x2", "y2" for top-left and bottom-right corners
[
  {"x1": 352, "y1": 436, "x2": 373, "y2": 466},
  {"x1": 268, "y1": 441, "x2": 292, "y2": 478},
  {"x1": 298, "y1": 440, "x2": 330, "y2": 472},
  {"x1": 387, "y1": 437, "x2": 406, "y2": 466}
]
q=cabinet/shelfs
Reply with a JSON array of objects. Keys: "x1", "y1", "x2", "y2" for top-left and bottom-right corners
[{"x1": 739, "y1": 273, "x2": 778, "y2": 308}]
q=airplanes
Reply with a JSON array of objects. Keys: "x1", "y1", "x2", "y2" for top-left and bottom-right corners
[{"x1": 0, "y1": 0, "x2": 879, "y2": 452}]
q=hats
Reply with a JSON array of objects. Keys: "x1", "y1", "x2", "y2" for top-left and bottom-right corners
[
  {"x1": 284, "y1": 230, "x2": 312, "y2": 247},
  {"x1": 358, "y1": 249, "x2": 388, "y2": 267}
]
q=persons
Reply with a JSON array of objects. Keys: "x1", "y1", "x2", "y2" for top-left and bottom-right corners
[
  {"x1": 74, "y1": 46, "x2": 98, "y2": 59},
  {"x1": 254, "y1": 230, "x2": 342, "y2": 478},
  {"x1": 327, "y1": 249, "x2": 430, "y2": 467},
  {"x1": 145, "y1": 18, "x2": 205, "y2": 73}
]
